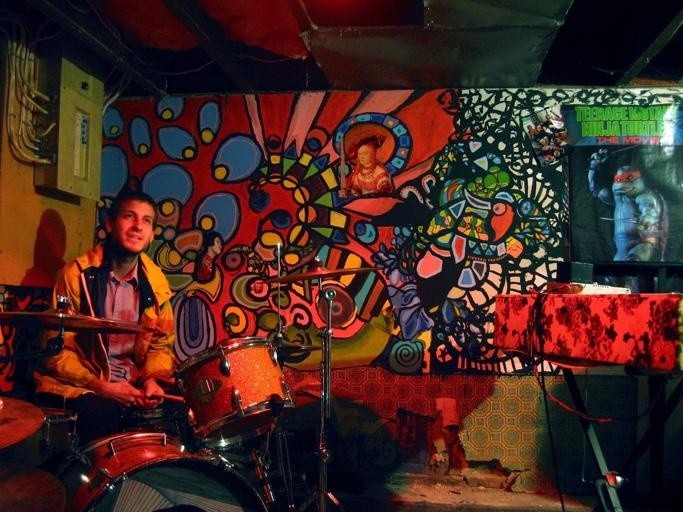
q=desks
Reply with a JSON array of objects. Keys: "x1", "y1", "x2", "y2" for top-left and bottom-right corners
[{"x1": 494, "y1": 293, "x2": 683, "y2": 512}]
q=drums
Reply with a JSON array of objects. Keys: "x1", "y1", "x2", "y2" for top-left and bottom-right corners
[
  {"x1": 174, "y1": 333, "x2": 289, "y2": 446},
  {"x1": 55, "y1": 428, "x2": 267, "y2": 510},
  {"x1": 28, "y1": 408, "x2": 77, "y2": 463}
]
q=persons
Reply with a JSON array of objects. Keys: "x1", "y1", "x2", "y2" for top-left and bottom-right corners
[
  {"x1": 339, "y1": 130, "x2": 392, "y2": 198},
  {"x1": 36, "y1": 184, "x2": 183, "y2": 413}
]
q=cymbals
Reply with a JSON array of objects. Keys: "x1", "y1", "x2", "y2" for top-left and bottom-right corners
[
  {"x1": 0, "y1": 308, "x2": 146, "y2": 333},
  {"x1": 259, "y1": 266, "x2": 385, "y2": 282},
  {"x1": 0, "y1": 396, "x2": 47, "y2": 447},
  {"x1": 272, "y1": 338, "x2": 321, "y2": 353}
]
